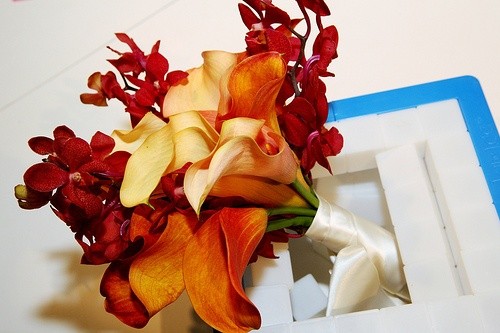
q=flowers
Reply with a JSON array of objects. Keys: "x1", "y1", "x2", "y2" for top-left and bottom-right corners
[{"x1": 13, "y1": 0, "x2": 413, "y2": 333}]
